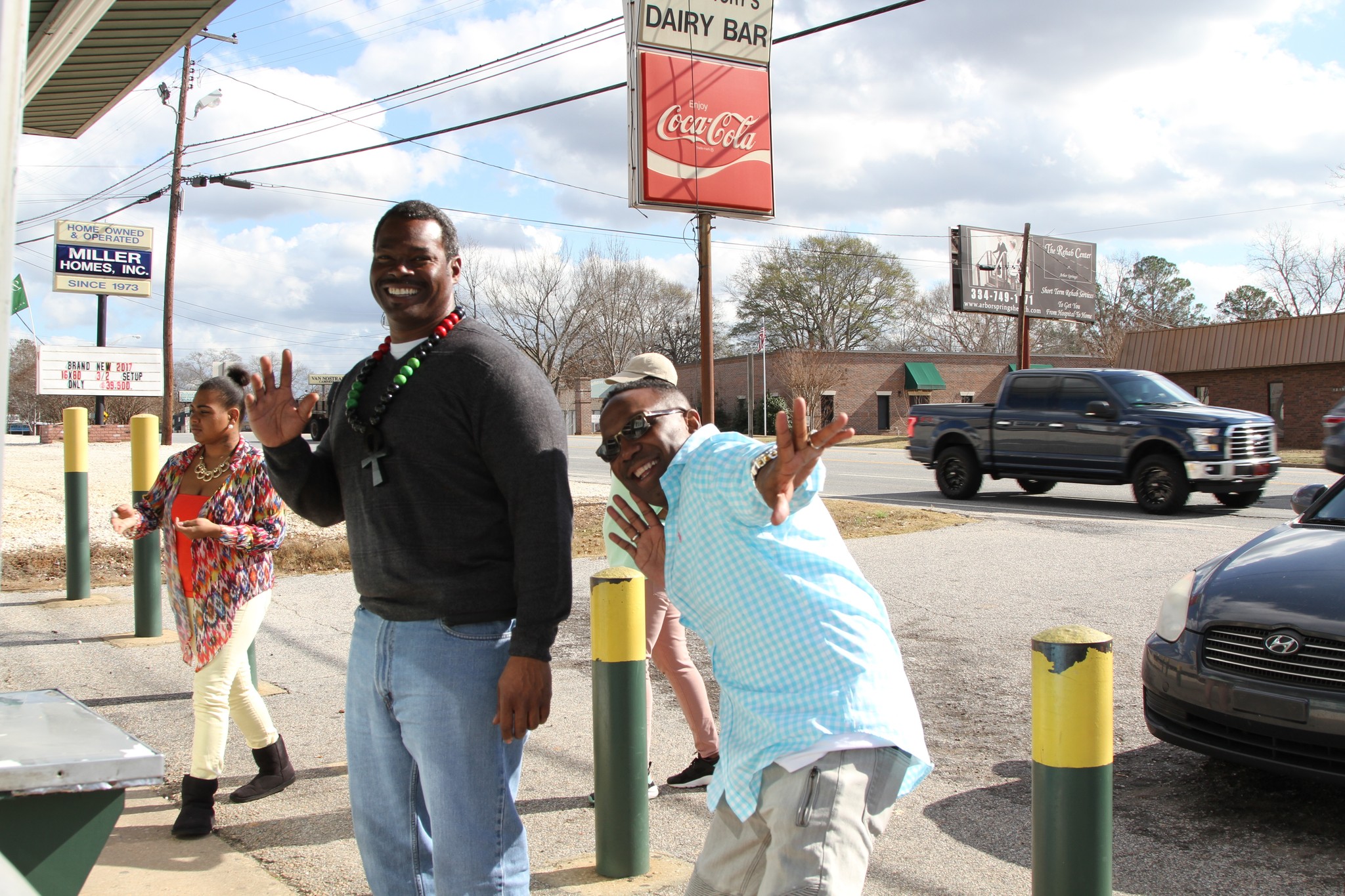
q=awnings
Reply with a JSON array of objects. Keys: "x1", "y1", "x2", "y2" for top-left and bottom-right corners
[
  {"x1": 904, "y1": 362, "x2": 946, "y2": 390},
  {"x1": 1008, "y1": 364, "x2": 1054, "y2": 372}
]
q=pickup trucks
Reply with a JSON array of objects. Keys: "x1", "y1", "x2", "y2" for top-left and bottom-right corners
[{"x1": 904, "y1": 367, "x2": 1283, "y2": 515}]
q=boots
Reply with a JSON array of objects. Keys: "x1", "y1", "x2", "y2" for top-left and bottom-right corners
[
  {"x1": 171, "y1": 774, "x2": 219, "y2": 839},
  {"x1": 229, "y1": 734, "x2": 296, "y2": 803}
]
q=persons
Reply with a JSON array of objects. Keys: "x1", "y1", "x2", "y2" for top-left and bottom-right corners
[
  {"x1": 244, "y1": 199, "x2": 574, "y2": 896},
  {"x1": 590, "y1": 352, "x2": 935, "y2": 896},
  {"x1": 109, "y1": 364, "x2": 297, "y2": 841}
]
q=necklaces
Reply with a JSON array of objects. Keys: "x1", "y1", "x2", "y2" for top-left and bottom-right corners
[
  {"x1": 194, "y1": 443, "x2": 238, "y2": 482},
  {"x1": 342, "y1": 303, "x2": 467, "y2": 438}
]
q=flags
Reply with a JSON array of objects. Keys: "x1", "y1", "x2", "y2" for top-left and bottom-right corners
[{"x1": 11, "y1": 273, "x2": 29, "y2": 316}]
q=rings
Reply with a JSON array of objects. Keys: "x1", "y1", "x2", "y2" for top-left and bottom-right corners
[
  {"x1": 631, "y1": 533, "x2": 640, "y2": 541},
  {"x1": 808, "y1": 428, "x2": 820, "y2": 450}
]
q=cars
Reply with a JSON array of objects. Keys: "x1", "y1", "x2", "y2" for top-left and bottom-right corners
[{"x1": 1141, "y1": 397, "x2": 1345, "y2": 784}]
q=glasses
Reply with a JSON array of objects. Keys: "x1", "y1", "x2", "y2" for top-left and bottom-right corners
[{"x1": 596, "y1": 408, "x2": 687, "y2": 463}]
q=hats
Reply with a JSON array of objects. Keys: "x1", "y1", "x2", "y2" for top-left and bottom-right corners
[{"x1": 604, "y1": 352, "x2": 678, "y2": 385}]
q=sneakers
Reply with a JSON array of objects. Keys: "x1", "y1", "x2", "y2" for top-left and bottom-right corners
[
  {"x1": 589, "y1": 761, "x2": 659, "y2": 803},
  {"x1": 666, "y1": 752, "x2": 719, "y2": 788}
]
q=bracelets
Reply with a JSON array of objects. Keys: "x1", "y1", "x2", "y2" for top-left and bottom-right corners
[
  {"x1": 122, "y1": 508, "x2": 139, "y2": 540},
  {"x1": 750, "y1": 449, "x2": 778, "y2": 494}
]
